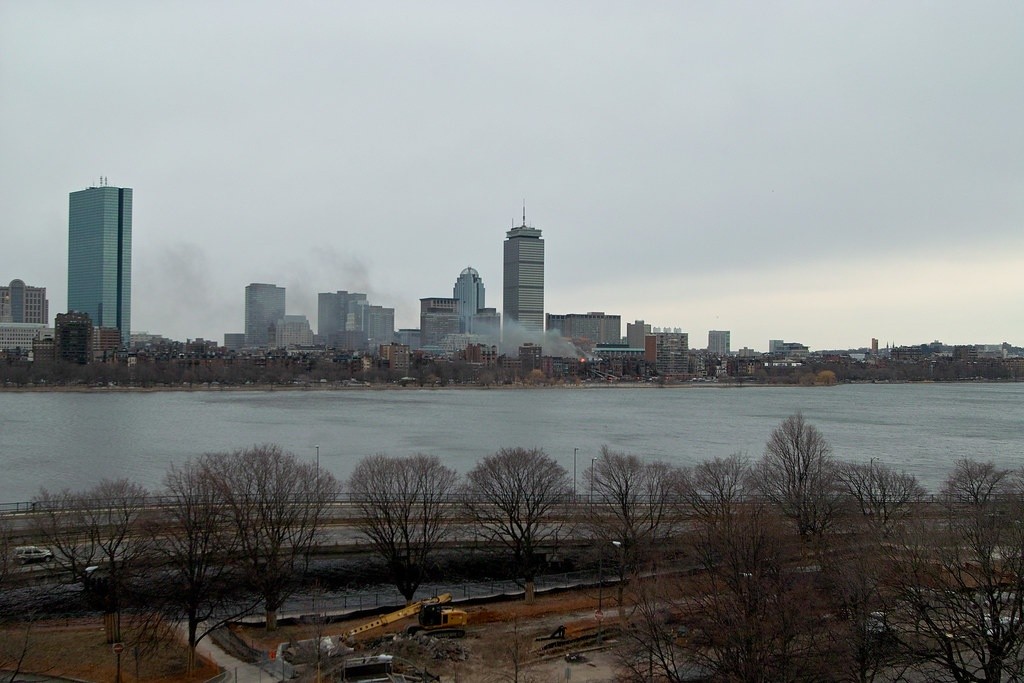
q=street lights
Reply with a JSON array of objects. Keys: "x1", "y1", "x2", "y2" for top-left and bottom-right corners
[
  {"x1": 315, "y1": 445, "x2": 319, "y2": 494},
  {"x1": 591, "y1": 458, "x2": 598, "y2": 508},
  {"x1": 574, "y1": 448, "x2": 580, "y2": 493}
]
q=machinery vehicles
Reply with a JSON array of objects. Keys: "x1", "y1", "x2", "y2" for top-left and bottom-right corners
[{"x1": 338, "y1": 592, "x2": 468, "y2": 652}]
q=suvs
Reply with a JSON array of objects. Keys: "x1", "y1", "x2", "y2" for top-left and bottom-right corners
[{"x1": 12, "y1": 546, "x2": 54, "y2": 564}]
